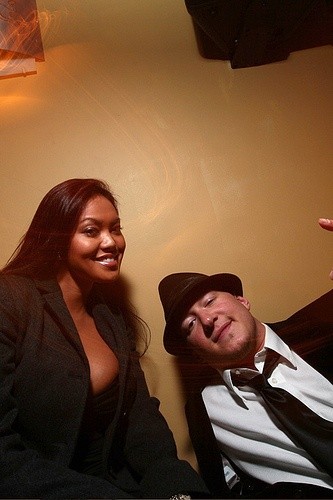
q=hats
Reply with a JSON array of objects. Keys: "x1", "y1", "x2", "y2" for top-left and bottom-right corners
[{"x1": 159, "y1": 273, "x2": 243, "y2": 363}]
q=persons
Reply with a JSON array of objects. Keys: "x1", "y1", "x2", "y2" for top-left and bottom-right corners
[
  {"x1": 0, "y1": 177, "x2": 212, "y2": 500},
  {"x1": 157, "y1": 216, "x2": 332, "y2": 499}
]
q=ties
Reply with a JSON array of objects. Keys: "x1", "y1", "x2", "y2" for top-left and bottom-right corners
[{"x1": 231, "y1": 348, "x2": 333, "y2": 480}]
